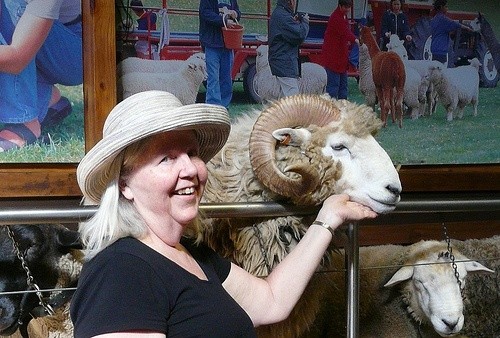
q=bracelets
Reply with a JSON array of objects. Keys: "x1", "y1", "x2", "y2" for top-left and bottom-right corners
[{"x1": 312, "y1": 221, "x2": 335, "y2": 238}]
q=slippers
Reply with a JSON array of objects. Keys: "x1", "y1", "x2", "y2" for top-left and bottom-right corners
[
  {"x1": 0, "y1": 123, "x2": 52, "y2": 152},
  {"x1": 40, "y1": 96, "x2": 72, "y2": 130}
]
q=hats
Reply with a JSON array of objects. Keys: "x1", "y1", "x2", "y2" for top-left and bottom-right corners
[{"x1": 77, "y1": 90, "x2": 231, "y2": 205}]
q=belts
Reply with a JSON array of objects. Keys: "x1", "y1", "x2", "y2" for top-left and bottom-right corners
[{"x1": 63, "y1": 15, "x2": 82, "y2": 26}]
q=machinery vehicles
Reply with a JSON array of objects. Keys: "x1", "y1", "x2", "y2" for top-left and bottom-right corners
[{"x1": 115, "y1": 0, "x2": 500, "y2": 101}]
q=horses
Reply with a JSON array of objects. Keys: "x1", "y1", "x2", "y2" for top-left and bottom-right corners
[{"x1": 350, "y1": 25, "x2": 406, "y2": 128}]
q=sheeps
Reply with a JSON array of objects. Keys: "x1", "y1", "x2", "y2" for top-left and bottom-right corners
[
  {"x1": 254, "y1": 37, "x2": 327, "y2": 102},
  {"x1": 0, "y1": 93, "x2": 500, "y2": 338},
  {"x1": 353, "y1": 33, "x2": 483, "y2": 121},
  {"x1": 119, "y1": 52, "x2": 208, "y2": 106}
]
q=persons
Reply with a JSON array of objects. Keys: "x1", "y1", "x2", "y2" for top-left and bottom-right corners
[
  {"x1": 321, "y1": 0, "x2": 360, "y2": 100},
  {"x1": 432, "y1": 0, "x2": 474, "y2": 62},
  {"x1": 380, "y1": 0, "x2": 412, "y2": 52},
  {"x1": 71, "y1": 90, "x2": 377, "y2": 338},
  {"x1": 199, "y1": 0, "x2": 241, "y2": 110},
  {"x1": 0, "y1": 0, "x2": 83, "y2": 153},
  {"x1": 268, "y1": 0, "x2": 309, "y2": 98},
  {"x1": 130, "y1": 0, "x2": 157, "y2": 39}
]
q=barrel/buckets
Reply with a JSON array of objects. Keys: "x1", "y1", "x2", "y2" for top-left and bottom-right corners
[{"x1": 222, "y1": 13, "x2": 244, "y2": 49}]
